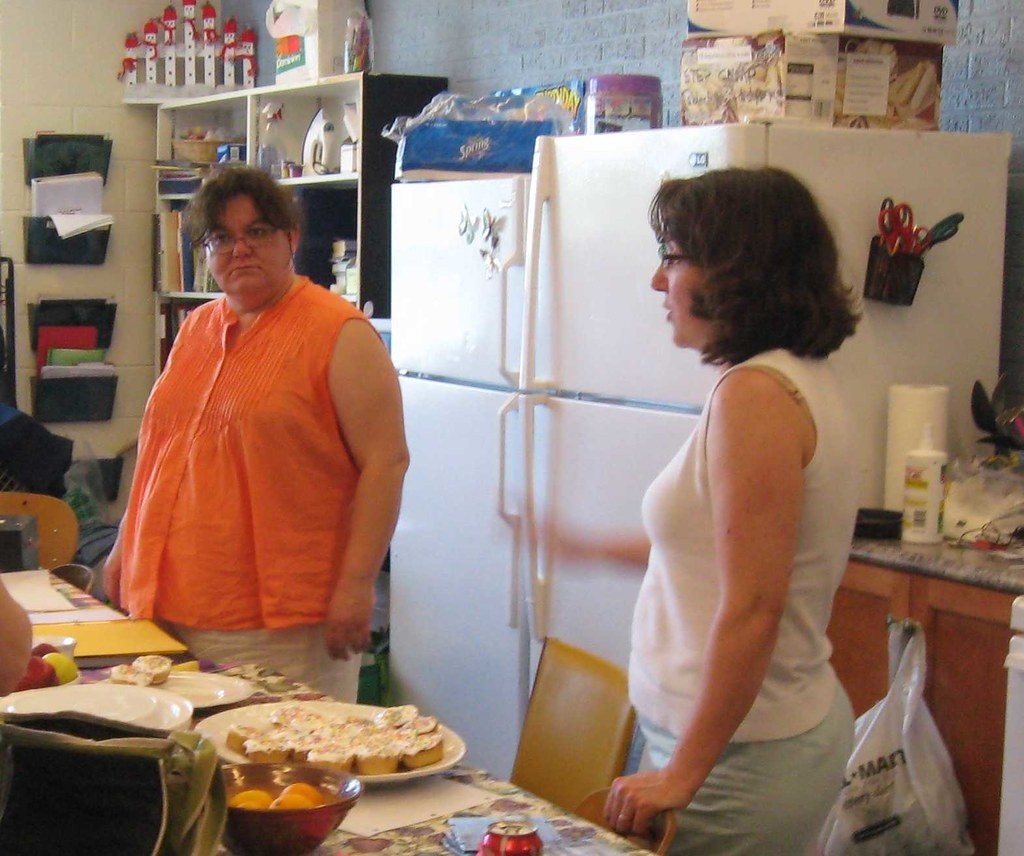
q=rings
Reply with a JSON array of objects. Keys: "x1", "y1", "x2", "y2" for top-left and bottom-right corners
[{"x1": 618, "y1": 814, "x2": 633, "y2": 821}]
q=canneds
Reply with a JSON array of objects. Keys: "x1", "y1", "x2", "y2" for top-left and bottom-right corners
[{"x1": 475, "y1": 821, "x2": 543, "y2": 856}]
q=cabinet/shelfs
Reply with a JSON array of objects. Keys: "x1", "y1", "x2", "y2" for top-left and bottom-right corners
[
  {"x1": 824, "y1": 560, "x2": 1018, "y2": 856},
  {"x1": 151, "y1": 71, "x2": 447, "y2": 380}
]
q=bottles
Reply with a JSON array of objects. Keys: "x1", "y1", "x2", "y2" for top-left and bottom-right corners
[{"x1": 901, "y1": 424, "x2": 947, "y2": 546}]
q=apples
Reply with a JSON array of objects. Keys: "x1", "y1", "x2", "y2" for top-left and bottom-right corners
[{"x1": 11, "y1": 644, "x2": 80, "y2": 694}]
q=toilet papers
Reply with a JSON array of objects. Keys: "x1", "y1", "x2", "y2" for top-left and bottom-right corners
[{"x1": 883, "y1": 384, "x2": 951, "y2": 513}]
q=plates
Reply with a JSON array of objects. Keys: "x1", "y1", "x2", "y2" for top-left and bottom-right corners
[
  {"x1": 101, "y1": 671, "x2": 254, "y2": 710},
  {"x1": 193, "y1": 700, "x2": 465, "y2": 784}
]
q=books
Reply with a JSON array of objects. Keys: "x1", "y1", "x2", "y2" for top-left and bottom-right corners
[{"x1": 152, "y1": 209, "x2": 222, "y2": 294}]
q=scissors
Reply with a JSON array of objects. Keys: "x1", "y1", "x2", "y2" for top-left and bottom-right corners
[{"x1": 878, "y1": 197, "x2": 963, "y2": 256}]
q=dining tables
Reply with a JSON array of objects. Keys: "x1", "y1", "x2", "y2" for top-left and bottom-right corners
[{"x1": 0, "y1": 569, "x2": 661, "y2": 856}]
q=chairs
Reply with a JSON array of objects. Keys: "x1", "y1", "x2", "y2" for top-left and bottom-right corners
[
  {"x1": 508, "y1": 637, "x2": 636, "y2": 814},
  {"x1": 50, "y1": 564, "x2": 96, "y2": 595},
  {"x1": 0, "y1": 492, "x2": 80, "y2": 571}
]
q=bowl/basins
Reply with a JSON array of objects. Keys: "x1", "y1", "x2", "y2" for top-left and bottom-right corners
[
  {"x1": 1, "y1": 684, "x2": 193, "y2": 734},
  {"x1": 59, "y1": 671, "x2": 81, "y2": 687},
  {"x1": 215, "y1": 762, "x2": 362, "y2": 856}
]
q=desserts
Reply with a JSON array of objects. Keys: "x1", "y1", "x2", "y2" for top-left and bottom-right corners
[
  {"x1": 112, "y1": 655, "x2": 171, "y2": 688},
  {"x1": 226, "y1": 703, "x2": 445, "y2": 777}
]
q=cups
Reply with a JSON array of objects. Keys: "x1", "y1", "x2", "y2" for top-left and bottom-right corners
[
  {"x1": 288, "y1": 164, "x2": 303, "y2": 177},
  {"x1": 30, "y1": 636, "x2": 77, "y2": 663},
  {"x1": 280, "y1": 159, "x2": 295, "y2": 179},
  {"x1": 270, "y1": 164, "x2": 281, "y2": 179},
  {"x1": 344, "y1": 16, "x2": 374, "y2": 73}
]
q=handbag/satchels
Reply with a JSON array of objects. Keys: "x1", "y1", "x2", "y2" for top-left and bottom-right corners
[
  {"x1": 0, "y1": 706, "x2": 231, "y2": 856},
  {"x1": 817, "y1": 616, "x2": 982, "y2": 856}
]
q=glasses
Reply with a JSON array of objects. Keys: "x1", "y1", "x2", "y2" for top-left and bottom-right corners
[
  {"x1": 655, "y1": 241, "x2": 697, "y2": 268},
  {"x1": 201, "y1": 223, "x2": 281, "y2": 255}
]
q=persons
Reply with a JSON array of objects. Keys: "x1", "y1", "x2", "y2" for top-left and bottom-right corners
[
  {"x1": 0, "y1": 578, "x2": 33, "y2": 698},
  {"x1": 600, "y1": 167, "x2": 858, "y2": 856},
  {"x1": 103, "y1": 166, "x2": 410, "y2": 704}
]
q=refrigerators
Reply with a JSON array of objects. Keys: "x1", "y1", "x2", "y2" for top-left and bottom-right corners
[{"x1": 391, "y1": 120, "x2": 1014, "y2": 788}]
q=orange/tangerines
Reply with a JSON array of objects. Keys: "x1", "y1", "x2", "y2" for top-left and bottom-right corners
[{"x1": 228, "y1": 784, "x2": 325, "y2": 809}]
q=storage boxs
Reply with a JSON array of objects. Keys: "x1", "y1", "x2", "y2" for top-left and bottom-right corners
[
  {"x1": 394, "y1": 120, "x2": 555, "y2": 178},
  {"x1": 688, "y1": 0, "x2": 960, "y2": 47},
  {"x1": 680, "y1": 29, "x2": 944, "y2": 131}
]
